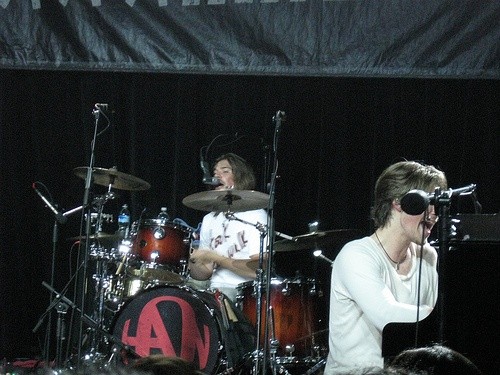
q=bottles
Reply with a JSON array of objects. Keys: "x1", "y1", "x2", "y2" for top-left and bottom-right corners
[
  {"x1": 118, "y1": 204, "x2": 130, "y2": 238},
  {"x1": 158, "y1": 207, "x2": 168, "y2": 219}
]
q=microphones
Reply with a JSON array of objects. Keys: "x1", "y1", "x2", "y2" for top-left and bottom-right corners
[
  {"x1": 275, "y1": 110, "x2": 286, "y2": 135},
  {"x1": 202, "y1": 176, "x2": 221, "y2": 185},
  {"x1": 401, "y1": 183, "x2": 477, "y2": 215},
  {"x1": 32, "y1": 183, "x2": 67, "y2": 225},
  {"x1": 96, "y1": 103, "x2": 112, "y2": 113}
]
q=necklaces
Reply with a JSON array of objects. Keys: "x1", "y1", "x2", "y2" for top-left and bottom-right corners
[
  {"x1": 374, "y1": 231, "x2": 408, "y2": 270},
  {"x1": 222, "y1": 209, "x2": 237, "y2": 232}
]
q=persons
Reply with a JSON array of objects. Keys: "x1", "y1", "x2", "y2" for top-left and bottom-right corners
[
  {"x1": 324, "y1": 161, "x2": 482, "y2": 375},
  {"x1": 126, "y1": 354, "x2": 210, "y2": 375},
  {"x1": 186, "y1": 153, "x2": 276, "y2": 302}
]
